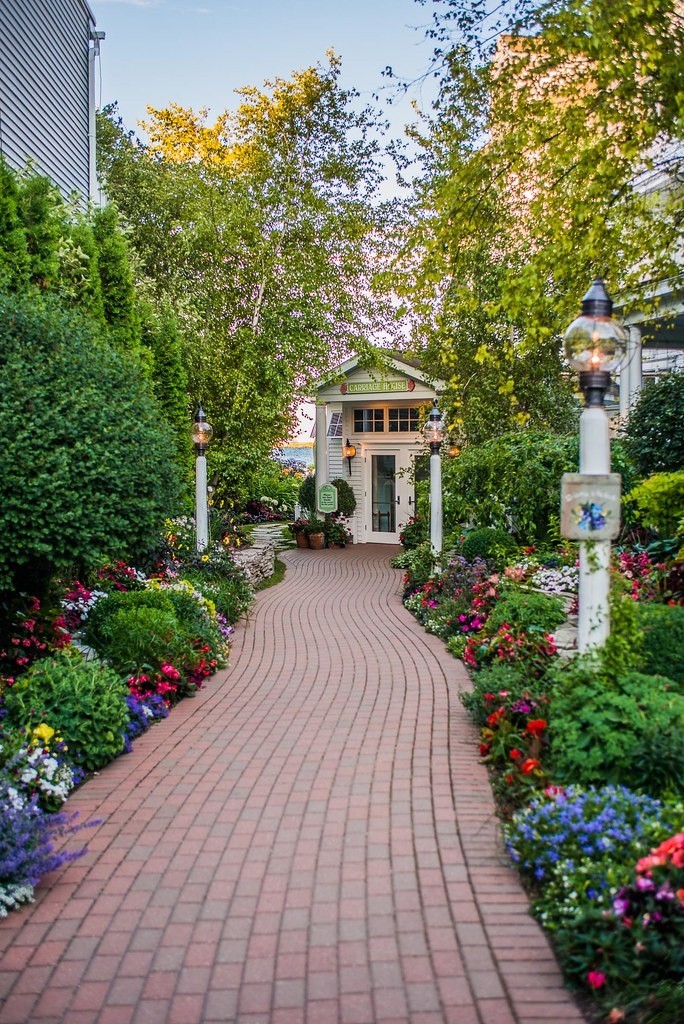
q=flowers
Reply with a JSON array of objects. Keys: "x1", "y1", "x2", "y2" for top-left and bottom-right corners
[{"x1": 287, "y1": 513, "x2": 354, "y2": 543}]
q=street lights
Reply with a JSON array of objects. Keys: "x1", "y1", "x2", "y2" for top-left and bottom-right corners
[
  {"x1": 420, "y1": 394, "x2": 446, "y2": 585},
  {"x1": 190, "y1": 402, "x2": 215, "y2": 554},
  {"x1": 561, "y1": 275, "x2": 633, "y2": 668}
]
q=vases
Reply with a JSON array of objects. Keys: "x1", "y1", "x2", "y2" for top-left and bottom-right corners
[
  {"x1": 339, "y1": 542, "x2": 346, "y2": 548},
  {"x1": 296, "y1": 529, "x2": 309, "y2": 548},
  {"x1": 310, "y1": 531, "x2": 325, "y2": 550},
  {"x1": 328, "y1": 541, "x2": 335, "y2": 550}
]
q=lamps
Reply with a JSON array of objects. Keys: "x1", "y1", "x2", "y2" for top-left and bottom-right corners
[
  {"x1": 448, "y1": 440, "x2": 459, "y2": 458},
  {"x1": 342, "y1": 438, "x2": 356, "y2": 476}
]
